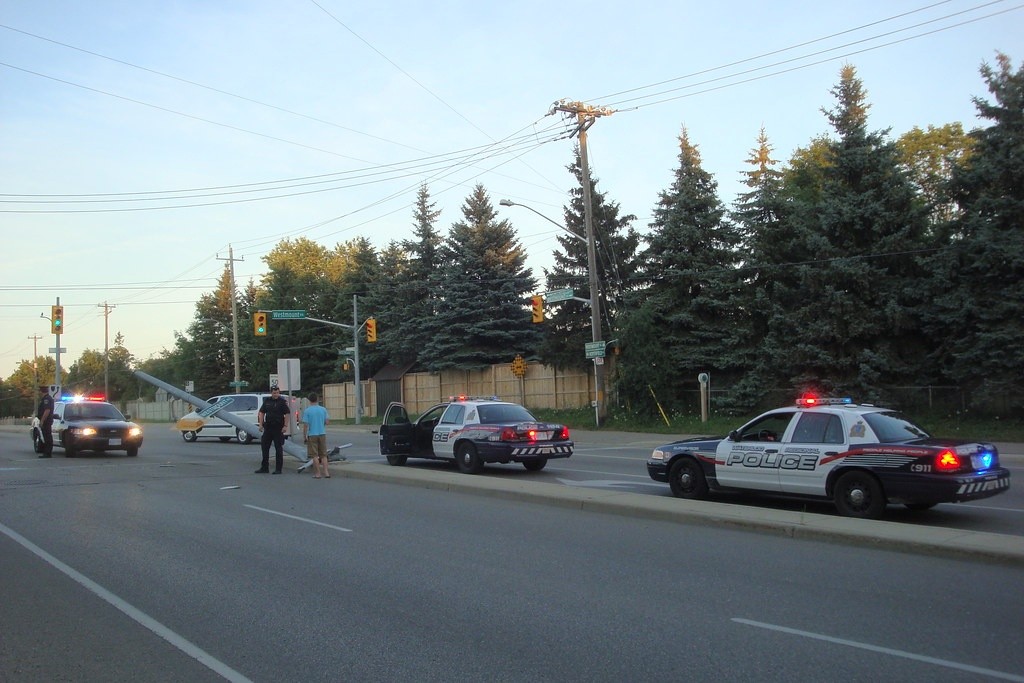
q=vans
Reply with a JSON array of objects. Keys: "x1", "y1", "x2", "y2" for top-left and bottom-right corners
[{"x1": 176, "y1": 392, "x2": 297, "y2": 443}]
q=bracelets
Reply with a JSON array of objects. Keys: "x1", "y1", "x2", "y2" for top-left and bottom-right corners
[{"x1": 284, "y1": 425, "x2": 287, "y2": 427}]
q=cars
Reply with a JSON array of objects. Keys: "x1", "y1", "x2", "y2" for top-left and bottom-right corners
[
  {"x1": 647, "y1": 396, "x2": 1013, "y2": 522},
  {"x1": 375, "y1": 395, "x2": 575, "y2": 474},
  {"x1": 33, "y1": 395, "x2": 143, "y2": 455}
]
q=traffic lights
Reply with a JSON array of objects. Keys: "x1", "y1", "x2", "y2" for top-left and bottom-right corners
[
  {"x1": 365, "y1": 318, "x2": 376, "y2": 343},
  {"x1": 253, "y1": 312, "x2": 267, "y2": 337},
  {"x1": 530, "y1": 294, "x2": 544, "y2": 324},
  {"x1": 52, "y1": 304, "x2": 63, "y2": 334}
]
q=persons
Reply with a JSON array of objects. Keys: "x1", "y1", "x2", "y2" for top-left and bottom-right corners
[
  {"x1": 38, "y1": 385, "x2": 55, "y2": 458},
  {"x1": 766, "y1": 433, "x2": 783, "y2": 442},
  {"x1": 301, "y1": 392, "x2": 331, "y2": 478},
  {"x1": 255, "y1": 385, "x2": 291, "y2": 475}
]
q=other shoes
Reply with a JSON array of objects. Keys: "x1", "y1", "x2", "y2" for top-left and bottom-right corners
[
  {"x1": 254, "y1": 468, "x2": 269, "y2": 473},
  {"x1": 272, "y1": 470, "x2": 281, "y2": 474}
]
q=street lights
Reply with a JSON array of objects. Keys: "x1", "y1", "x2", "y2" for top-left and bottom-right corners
[{"x1": 498, "y1": 102, "x2": 610, "y2": 424}]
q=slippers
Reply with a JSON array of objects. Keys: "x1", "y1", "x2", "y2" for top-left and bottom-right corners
[
  {"x1": 312, "y1": 475, "x2": 322, "y2": 478},
  {"x1": 324, "y1": 475, "x2": 331, "y2": 478}
]
